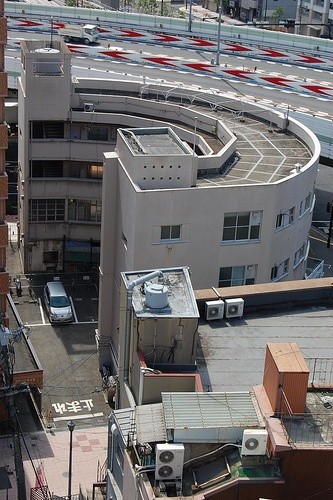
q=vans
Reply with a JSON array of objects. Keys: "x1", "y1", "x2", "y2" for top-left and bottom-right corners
[{"x1": 43, "y1": 281, "x2": 74, "y2": 323}]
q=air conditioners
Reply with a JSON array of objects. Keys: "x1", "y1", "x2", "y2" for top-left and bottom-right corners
[
  {"x1": 205, "y1": 300, "x2": 224, "y2": 320},
  {"x1": 155, "y1": 443, "x2": 185, "y2": 480},
  {"x1": 241, "y1": 429, "x2": 268, "y2": 457},
  {"x1": 225, "y1": 298, "x2": 244, "y2": 318}
]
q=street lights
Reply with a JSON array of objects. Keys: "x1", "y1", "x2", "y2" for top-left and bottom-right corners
[
  {"x1": 215, "y1": 6, "x2": 222, "y2": 65},
  {"x1": 64, "y1": 422, "x2": 76, "y2": 498}
]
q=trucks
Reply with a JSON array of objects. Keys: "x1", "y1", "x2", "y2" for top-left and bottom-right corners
[{"x1": 58, "y1": 21, "x2": 99, "y2": 44}]
